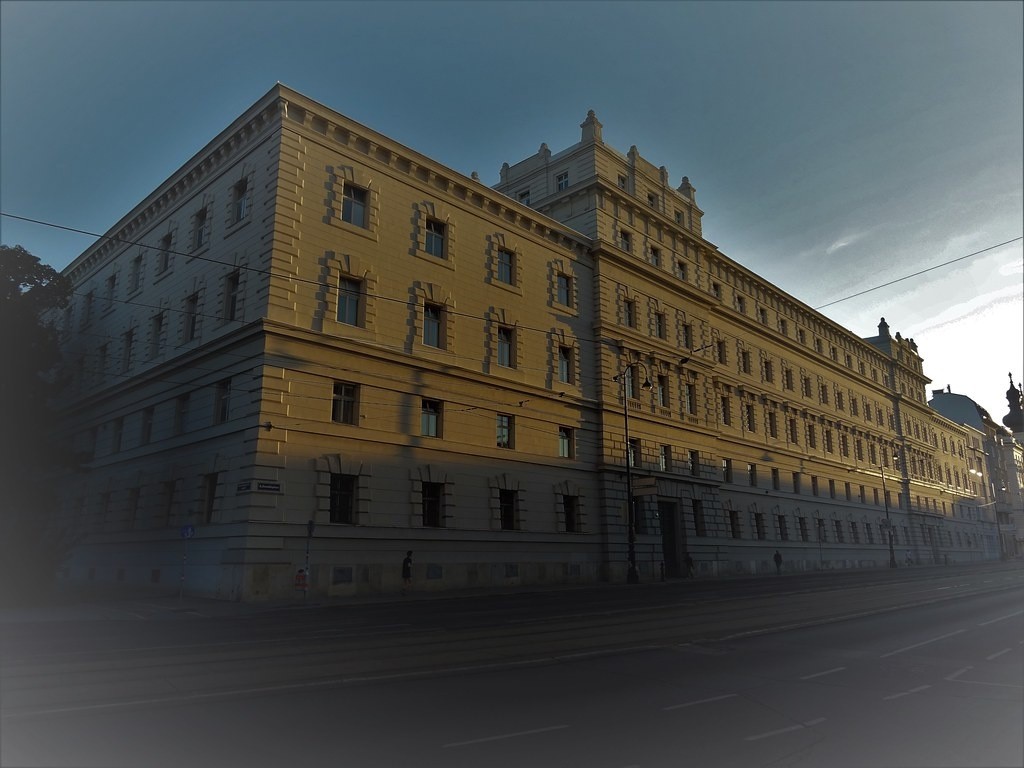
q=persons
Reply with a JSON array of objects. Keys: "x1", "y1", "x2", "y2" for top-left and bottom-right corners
[
  {"x1": 906, "y1": 550, "x2": 912, "y2": 565},
  {"x1": 401, "y1": 549, "x2": 416, "y2": 596},
  {"x1": 687, "y1": 554, "x2": 695, "y2": 579},
  {"x1": 295, "y1": 568, "x2": 304, "y2": 593},
  {"x1": 774, "y1": 550, "x2": 781, "y2": 575}
]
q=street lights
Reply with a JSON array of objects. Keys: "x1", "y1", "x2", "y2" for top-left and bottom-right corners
[
  {"x1": 622, "y1": 362, "x2": 652, "y2": 583},
  {"x1": 879, "y1": 440, "x2": 900, "y2": 569}
]
